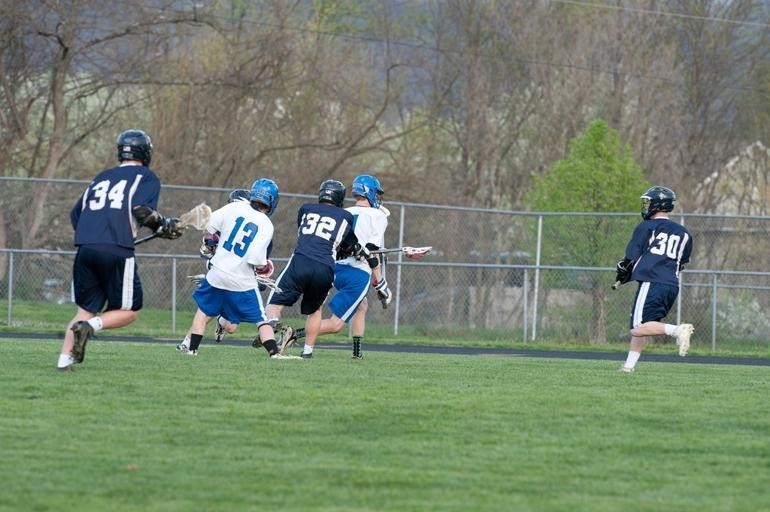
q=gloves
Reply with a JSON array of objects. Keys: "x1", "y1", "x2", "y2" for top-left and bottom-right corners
[
  {"x1": 254, "y1": 260, "x2": 274, "y2": 291},
  {"x1": 345, "y1": 243, "x2": 370, "y2": 261},
  {"x1": 372, "y1": 277, "x2": 392, "y2": 309},
  {"x1": 142, "y1": 210, "x2": 185, "y2": 239},
  {"x1": 200, "y1": 234, "x2": 219, "y2": 259},
  {"x1": 616, "y1": 261, "x2": 631, "y2": 283}
]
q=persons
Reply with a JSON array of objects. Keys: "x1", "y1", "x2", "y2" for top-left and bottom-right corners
[
  {"x1": 250, "y1": 179, "x2": 370, "y2": 359},
  {"x1": 56, "y1": 127, "x2": 184, "y2": 372},
  {"x1": 613, "y1": 185, "x2": 695, "y2": 375},
  {"x1": 175, "y1": 178, "x2": 289, "y2": 357},
  {"x1": 202, "y1": 190, "x2": 277, "y2": 344},
  {"x1": 278, "y1": 172, "x2": 397, "y2": 360}
]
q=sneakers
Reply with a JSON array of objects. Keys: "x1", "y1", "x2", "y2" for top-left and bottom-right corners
[
  {"x1": 252, "y1": 320, "x2": 302, "y2": 360},
  {"x1": 176, "y1": 343, "x2": 197, "y2": 356},
  {"x1": 215, "y1": 314, "x2": 225, "y2": 342},
  {"x1": 70, "y1": 321, "x2": 93, "y2": 362},
  {"x1": 673, "y1": 324, "x2": 695, "y2": 357}
]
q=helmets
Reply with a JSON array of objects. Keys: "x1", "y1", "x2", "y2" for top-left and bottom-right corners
[
  {"x1": 319, "y1": 180, "x2": 346, "y2": 208},
  {"x1": 250, "y1": 178, "x2": 280, "y2": 216},
  {"x1": 640, "y1": 186, "x2": 676, "y2": 220},
  {"x1": 116, "y1": 129, "x2": 154, "y2": 164},
  {"x1": 352, "y1": 174, "x2": 385, "y2": 208},
  {"x1": 228, "y1": 189, "x2": 251, "y2": 203}
]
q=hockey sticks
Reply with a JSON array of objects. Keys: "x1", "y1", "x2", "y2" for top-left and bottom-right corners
[
  {"x1": 134, "y1": 203, "x2": 213, "y2": 245},
  {"x1": 369, "y1": 246, "x2": 432, "y2": 258}
]
